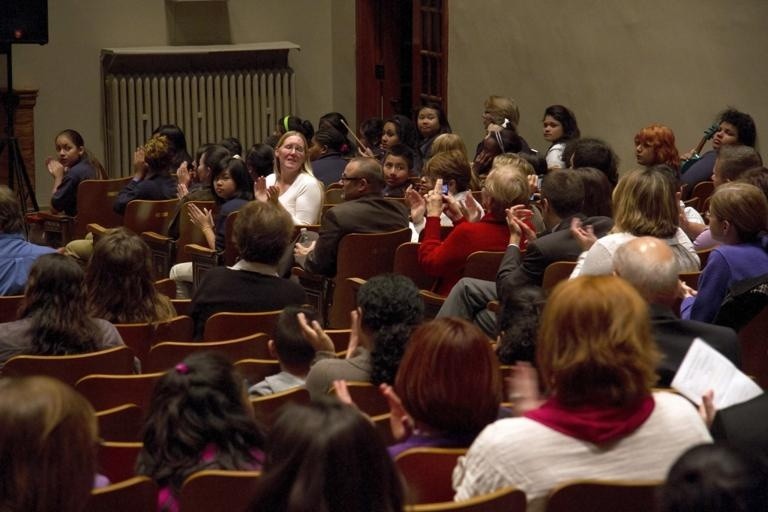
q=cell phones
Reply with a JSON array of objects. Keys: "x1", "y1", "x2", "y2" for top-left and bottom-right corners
[{"x1": 441, "y1": 182, "x2": 449, "y2": 203}]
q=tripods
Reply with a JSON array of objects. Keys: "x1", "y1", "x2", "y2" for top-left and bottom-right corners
[{"x1": 0, "y1": 44, "x2": 39, "y2": 242}]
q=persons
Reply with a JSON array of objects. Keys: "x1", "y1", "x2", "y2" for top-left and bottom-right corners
[{"x1": 1, "y1": 93, "x2": 767, "y2": 512}]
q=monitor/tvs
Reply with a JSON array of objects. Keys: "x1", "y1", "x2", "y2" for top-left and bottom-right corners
[{"x1": 0, "y1": 0, "x2": 49, "y2": 45}]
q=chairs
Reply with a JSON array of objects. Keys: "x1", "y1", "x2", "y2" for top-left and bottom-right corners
[
  {"x1": 546, "y1": 479, "x2": 669, "y2": 512},
  {"x1": 403, "y1": 487, "x2": 528, "y2": 511}
]
